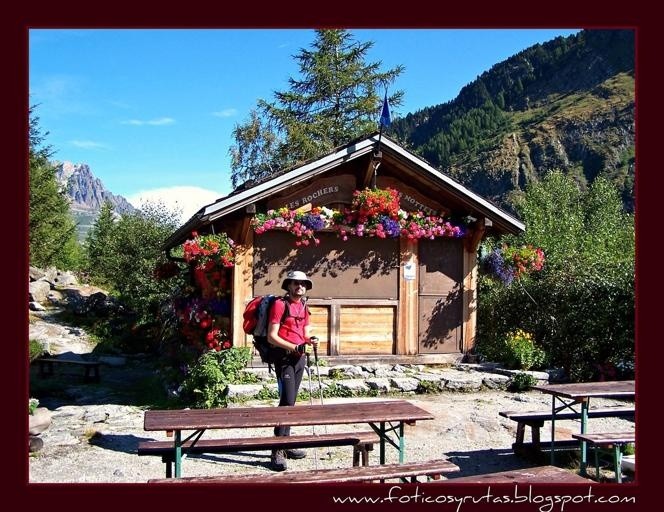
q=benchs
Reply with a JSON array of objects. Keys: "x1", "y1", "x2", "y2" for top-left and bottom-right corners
[
  {"x1": 500, "y1": 406, "x2": 634, "y2": 483},
  {"x1": 134, "y1": 431, "x2": 461, "y2": 482}
]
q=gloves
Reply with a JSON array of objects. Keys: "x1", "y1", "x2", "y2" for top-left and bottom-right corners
[
  {"x1": 295, "y1": 341, "x2": 313, "y2": 355},
  {"x1": 311, "y1": 336, "x2": 320, "y2": 348}
]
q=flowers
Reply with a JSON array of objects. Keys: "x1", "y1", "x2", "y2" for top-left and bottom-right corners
[
  {"x1": 479, "y1": 239, "x2": 545, "y2": 285},
  {"x1": 173, "y1": 236, "x2": 241, "y2": 354},
  {"x1": 247, "y1": 186, "x2": 466, "y2": 250}
]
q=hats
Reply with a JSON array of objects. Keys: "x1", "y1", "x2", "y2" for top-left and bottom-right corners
[{"x1": 281, "y1": 270, "x2": 313, "y2": 292}]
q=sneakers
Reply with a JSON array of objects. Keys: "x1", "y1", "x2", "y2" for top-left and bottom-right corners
[
  {"x1": 271, "y1": 450, "x2": 287, "y2": 471},
  {"x1": 285, "y1": 448, "x2": 307, "y2": 459}
]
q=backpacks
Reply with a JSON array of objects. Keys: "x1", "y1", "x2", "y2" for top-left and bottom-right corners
[{"x1": 242, "y1": 293, "x2": 306, "y2": 364}]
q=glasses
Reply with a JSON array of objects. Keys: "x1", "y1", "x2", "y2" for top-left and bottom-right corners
[{"x1": 295, "y1": 280, "x2": 307, "y2": 285}]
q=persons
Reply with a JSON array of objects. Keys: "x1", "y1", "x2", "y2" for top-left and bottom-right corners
[{"x1": 267, "y1": 268, "x2": 321, "y2": 472}]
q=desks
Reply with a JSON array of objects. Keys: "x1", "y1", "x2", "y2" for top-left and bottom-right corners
[
  {"x1": 144, "y1": 400, "x2": 435, "y2": 484},
  {"x1": 531, "y1": 381, "x2": 635, "y2": 469}
]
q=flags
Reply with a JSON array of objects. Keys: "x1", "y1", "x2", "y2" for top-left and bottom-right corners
[{"x1": 379, "y1": 89, "x2": 393, "y2": 133}]
